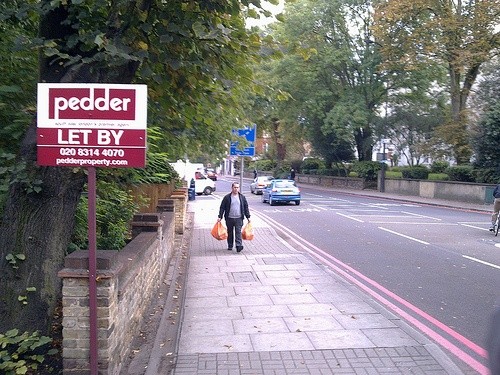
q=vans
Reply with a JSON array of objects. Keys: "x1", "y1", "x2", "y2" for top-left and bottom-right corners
[{"x1": 176, "y1": 170, "x2": 216, "y2": 194}]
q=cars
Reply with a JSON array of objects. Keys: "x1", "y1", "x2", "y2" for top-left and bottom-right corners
[
  {"x1": 206, "y1": 171, "x2": 218, "y2": 181},
  {"x1": 250, "y1": 176, "x2": 276, "y2": 195},
  {"x1": 262, "y1": 179, "x2": 301, "y2": 205}
]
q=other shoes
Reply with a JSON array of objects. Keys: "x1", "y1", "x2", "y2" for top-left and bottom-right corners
[
  {"x1": 236, "y1": 245, "x2": 243, "y2": 252},
  {"x1": 489, "y1": 227, "x2": 493, "y2": 231},
  {"x1": 228, "y1": 244, "x2": 233, "y2": 250}
]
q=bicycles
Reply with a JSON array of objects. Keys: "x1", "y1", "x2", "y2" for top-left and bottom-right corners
[{"x1": 491, "y1": 199, "x2": 500, "y2": 236}]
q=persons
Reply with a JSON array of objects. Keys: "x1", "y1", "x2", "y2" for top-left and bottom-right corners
[
  {"x1": 217, "y1": 181, "x2": 252, "y2": 252},
  {"x1": 253, "y1": 167, "x2": 259, "y2": 179},
  {"x1": 290, "y1": 168, "x2": 296, "y2": 180},
  {"x1": 489, "y1": 184, "x2": 500, "y2": 232},
  {"x1": 195, "y1": 172, "x2": 200, "y2": 179}
]
